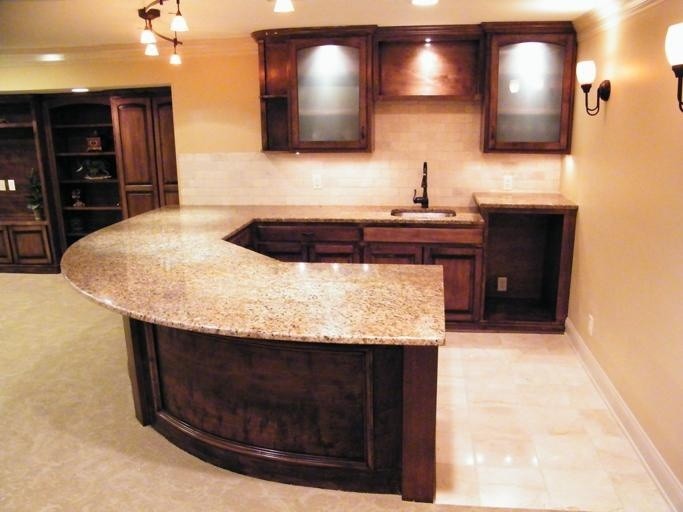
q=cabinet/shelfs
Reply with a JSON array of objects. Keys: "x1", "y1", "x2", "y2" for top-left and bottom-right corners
[
  {"x1": 56, "y1": 90, "x2": 123, "y2": 276},
  {"x1": 0, "y1": 90, "x2": 55, "y2": 273},
  {"x1": 111, "y1": 86, "x2": 178, "y2": 221},
  {"x1": 258, "y1": 226, "x2": 479, "y2": 330},
  {"x1": 252, "y1": 22, "x2": 577, "y2": 153}
]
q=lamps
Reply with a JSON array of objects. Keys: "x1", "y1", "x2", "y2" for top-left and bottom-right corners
[
  {"x1": 664, "y1": 22, "x2": 683, "y2": 112},
  {"x1": 574, "y1": 60, "x2": 612, "y2": 117},
  {"x1": 137, "y1": 1, "x2": 189, "y2": 65}
]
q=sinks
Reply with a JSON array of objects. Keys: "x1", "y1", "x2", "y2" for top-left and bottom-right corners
[{"x1": 391, "y1": 209, "x2": 456, "y2": 219}]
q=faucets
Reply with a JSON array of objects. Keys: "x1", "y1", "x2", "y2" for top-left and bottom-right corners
[{"x1": 412, "y1": 161, "x2": 428, "y2": 208}]
